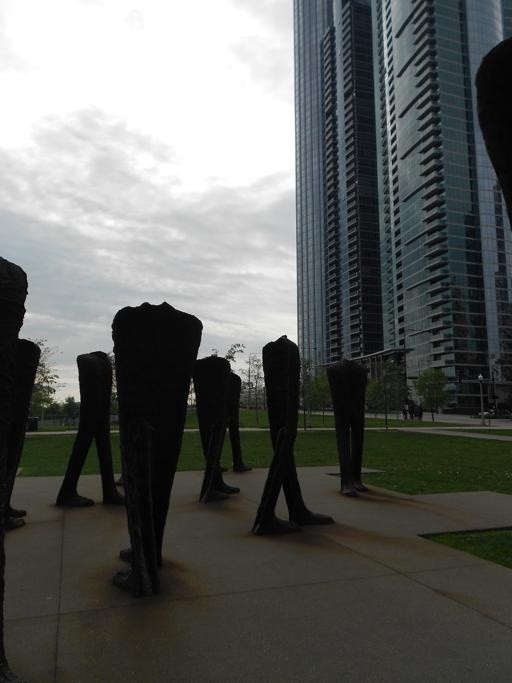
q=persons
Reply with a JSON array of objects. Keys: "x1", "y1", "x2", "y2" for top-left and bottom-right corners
[
  {"x1": 330, "y1": 359, "x2": 369, "y2": 497},
  {"x1": 55, "y1": 351, "x2": 125, "y2": 508},
  {"x1": 252, "y1": 335, "x2": 335, "y2": 535},
  {"x1": 404, "y1": 398, "x2": 422, "y2": 420},
  {"x1": 194, "y1": 354, "x2": 252, "y2": 503},
  {"x1": 112, "y1": 302, "x2": 203, "y2": 597},
  {"x1": 1, "y1": 257, "x2": 40, "y2": 683}
]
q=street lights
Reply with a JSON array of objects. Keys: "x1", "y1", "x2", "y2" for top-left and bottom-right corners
[{"x1": 478, "y1": 373, "x2": 485, "y2": 426}]
